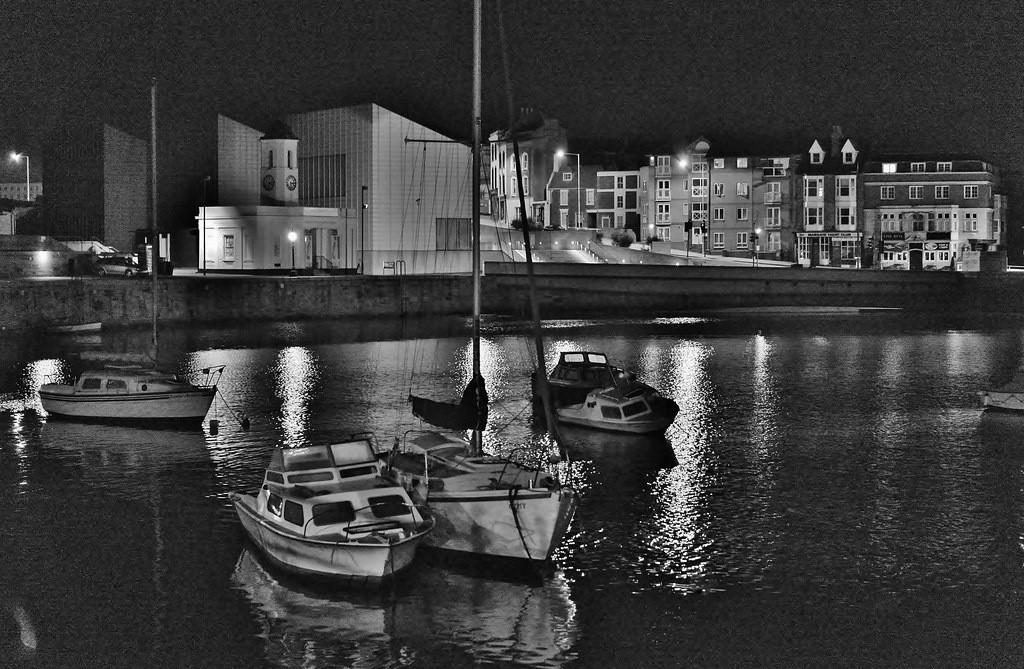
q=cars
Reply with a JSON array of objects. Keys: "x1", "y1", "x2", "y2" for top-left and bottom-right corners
[{"x1": 92, "y1": 257, "x2": 149, "y2": 279}]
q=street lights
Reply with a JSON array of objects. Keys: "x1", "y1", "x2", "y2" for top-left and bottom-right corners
[
  {"x1": 288, "y1": 232, "x2": 298, "y2": 276},
  {"x1": 755, "y1": 228, "x2": 761, "y2": 267},
  {"x1": 557, "y1": 150, "x2": 580, "y2": 229},
  {"x1": 9, "y1": 153, "x2": 30, "y2": 200},
  {"x1": 705, "y1": 147, "x2": 714, "y2": 253},
  {"x1": 361, "y1": 186, "x2": 369, "y2": 275}
]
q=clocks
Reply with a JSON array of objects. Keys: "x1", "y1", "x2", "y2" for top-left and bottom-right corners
[
  {"x1": 286, "y1": 175, "x2": 297, "y2": 191},
  {"x1": 263, "y1": 175, "x2": 275, "y2": 191}
]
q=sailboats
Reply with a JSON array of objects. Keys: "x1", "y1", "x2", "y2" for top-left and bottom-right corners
[
  {"x1": 39, "y1": 74, "x2": 227, "y2": 424},
  {"x1": 345, "y1": 0, "x2": 579, "y2": 571}
]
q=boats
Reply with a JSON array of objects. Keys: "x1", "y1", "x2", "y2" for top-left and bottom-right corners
[
  {"x1": 228, "y1": 432, "x2": 436, "y2": 589},
  {"x1": 531, "y1": 352, "x2": 680, "y2": 437}
]
